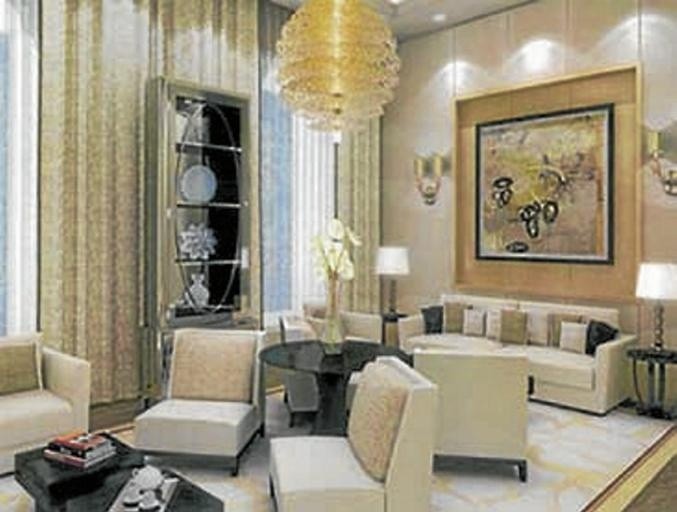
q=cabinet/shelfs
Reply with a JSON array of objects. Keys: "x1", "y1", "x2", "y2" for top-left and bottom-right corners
[{"x1": 140, "y1": 77, "x2": 256, "y2": 404}]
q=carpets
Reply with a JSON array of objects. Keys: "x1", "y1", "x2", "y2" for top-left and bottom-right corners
[{"x1": 0, "y1": 384, "x2": 677, "y2": 511}]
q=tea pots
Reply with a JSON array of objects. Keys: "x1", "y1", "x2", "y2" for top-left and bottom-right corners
[{"x1": 132, "y1": 464, "x2": 168, "y2": 491}]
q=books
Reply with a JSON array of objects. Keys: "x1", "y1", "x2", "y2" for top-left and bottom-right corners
[{"x1": 42, "y1": 431, "x2": 117, "y2": 470}]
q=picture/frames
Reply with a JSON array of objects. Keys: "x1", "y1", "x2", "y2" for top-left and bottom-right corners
[{"x1": 473, "y1": 101, "x2": 616, "y2": 267}]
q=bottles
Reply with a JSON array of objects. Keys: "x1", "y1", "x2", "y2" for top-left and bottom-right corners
[{"x1": 184, "y1": 272, "x2": 210, "y2": 310}]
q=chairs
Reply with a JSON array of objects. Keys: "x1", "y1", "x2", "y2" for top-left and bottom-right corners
[
  {"x1": 410, "y1": 348, "x2": 536, "y2": 488},
  {"x1": 0, "y1": 333, "x2": 93, "y2": 481},
  {"x1": 128, "y1": 324, "x2": 270, "y2": 478},
  {"x1": 270, "y1": 350, "x2": 440, "y2": 510}
]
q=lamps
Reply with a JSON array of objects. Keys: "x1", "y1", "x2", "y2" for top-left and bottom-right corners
[
  {"x1": 274, "y1": 1, "x2": 403, "y2": 149},
  {"x1": 410, "y1": 152, "x2": 449, "y2": 205},
  {"x1": 633, "y1": 259, "x2": 676, "y2": 353},
  {"x1": 638, "y1": 123, "x2": 675, "y2": 198},
  {"x1": 373, "y1": 246, "x2": 412, "y2": 318}
]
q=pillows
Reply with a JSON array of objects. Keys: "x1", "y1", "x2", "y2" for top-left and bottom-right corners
[
  {"x1": 0, "y1": 337, "x2": 44, "y2": 396},
  {"x1": 301, "y1": 298, "x2": 328, "y2": 322},
  {"x1": 419, "y1": 301, "x2": 619, "y2": 360},
  {"x1": 345, "y1": 362, "x2": 410, "y2": 484},
  {"x1": 169, "y1": 330, "x2": 259, "y2": 405}
]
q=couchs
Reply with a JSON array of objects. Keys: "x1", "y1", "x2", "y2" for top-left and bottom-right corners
[
  {"x1": 394, "y1": 291, "x2": 640, "y2": 416},
  {"x1": 263, "y1": 302, "x2": 384, "y2": 386}
]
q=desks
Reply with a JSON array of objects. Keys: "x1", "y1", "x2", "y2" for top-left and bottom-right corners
[
  {"x1": 380, "y1": 315, "x2": 404, "y2": 351},
  {"x1": 257, "y1": 338, "x2": 414, "y2": 440},
  {"x1": 626, "y1": 347, "x2": 676, "y2": 420},
  {"x1": 12, "y1": 429, "x2": 223, "y2": 509}
]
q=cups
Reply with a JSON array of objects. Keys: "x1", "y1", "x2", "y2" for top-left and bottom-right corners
[{"x1": 122, "y1": 485, "x2": 159, "y2": 509}]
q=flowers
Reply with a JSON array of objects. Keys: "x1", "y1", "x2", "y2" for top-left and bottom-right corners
[{"x1": 304, "y1": 218, "x2": 373, "y2": 344}]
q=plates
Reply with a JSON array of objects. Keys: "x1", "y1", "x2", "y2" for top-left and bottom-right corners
[
  {"x1": 105, "y1": 477, "x2": 181, "y2": 512},
  {"x1": 180, "y1": 164, "x2": 218, "y2": 203}
]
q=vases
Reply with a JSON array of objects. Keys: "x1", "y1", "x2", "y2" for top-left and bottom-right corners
[{"x1": 316, "y1": 283, "x2": 346, "y2": 356}]
